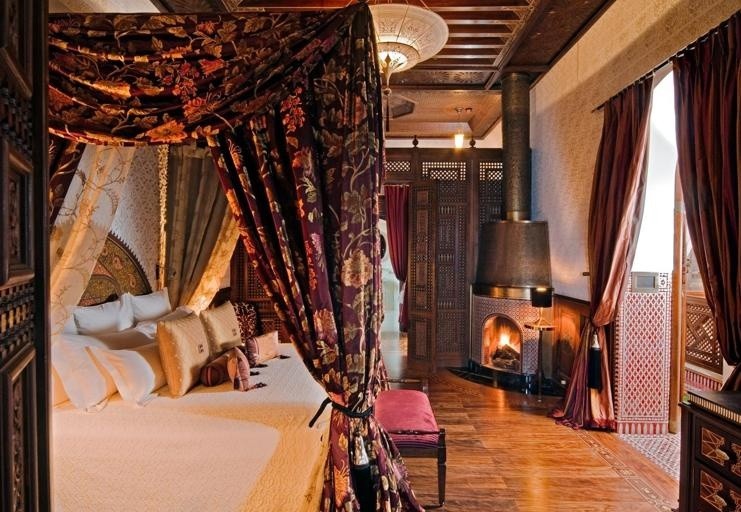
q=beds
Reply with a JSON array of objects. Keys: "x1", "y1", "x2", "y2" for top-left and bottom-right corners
[{"x1": 45, "y1": 220, "x2": 333, "y2": 508}]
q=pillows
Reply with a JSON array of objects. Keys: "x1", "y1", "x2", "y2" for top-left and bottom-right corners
[{"x1": 50, "y1": 287, "x2": 278, "y2": 416}]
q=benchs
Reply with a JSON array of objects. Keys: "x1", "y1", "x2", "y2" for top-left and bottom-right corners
[{"x1": 370, "y1": 378, "x2": 446, "y2": 506}]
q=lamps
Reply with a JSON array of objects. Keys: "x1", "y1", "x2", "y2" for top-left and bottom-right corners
[{"x1": 367, "y1": 1, "x2": 451, "y2": 95}]
